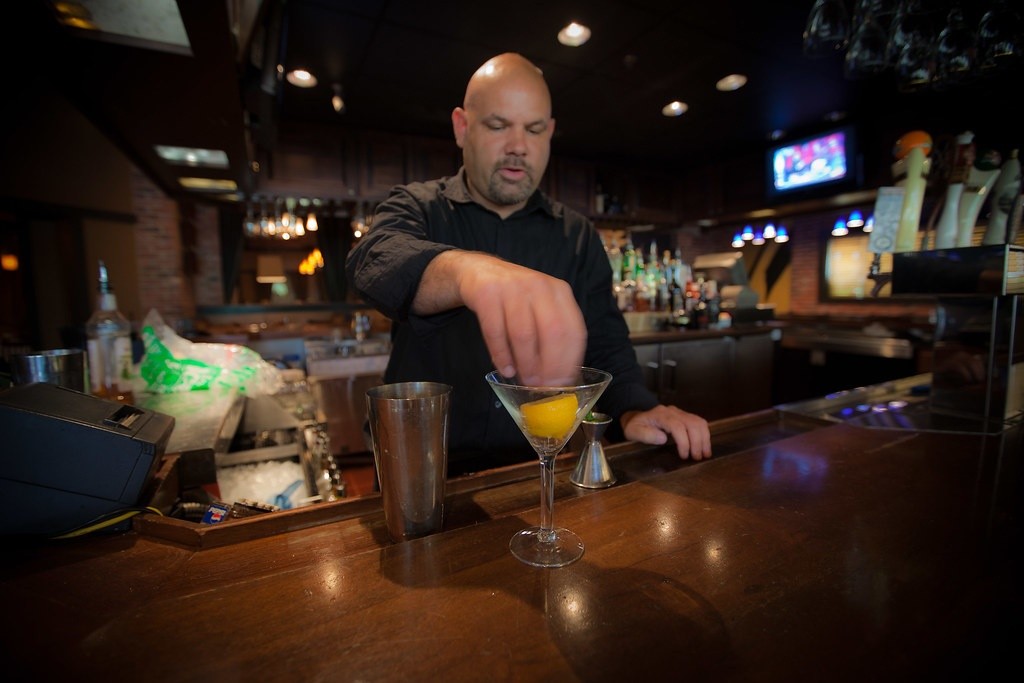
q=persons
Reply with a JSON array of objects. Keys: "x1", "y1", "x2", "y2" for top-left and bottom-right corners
[{"x1": 341, "y1": 54, "x2": 714, "y2": 495}]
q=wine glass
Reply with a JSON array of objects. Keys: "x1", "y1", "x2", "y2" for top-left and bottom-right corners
[{"x1": 485, "y1": 367, "x2": 613, "y2": 568}]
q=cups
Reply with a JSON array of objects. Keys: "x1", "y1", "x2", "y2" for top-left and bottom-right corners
[
  {"x1": 23, "y1": 350, "x2": 85, "y2": 393},
  {"x1": 365, "y1": 381, "x2": 453, "y2": 543}
]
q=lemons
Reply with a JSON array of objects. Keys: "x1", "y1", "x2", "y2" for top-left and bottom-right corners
[{"x1": 521, "y1": 393, "x2": 578, "y2": 440}]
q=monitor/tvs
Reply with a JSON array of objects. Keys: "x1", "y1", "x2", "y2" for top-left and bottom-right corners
[{"x1": 763, "y1": 123, "x2": 865, "y2": 201}]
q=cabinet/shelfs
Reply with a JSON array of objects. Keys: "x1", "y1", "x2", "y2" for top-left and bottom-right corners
[{"x1": 633, "y1": 334, "x2": 773, "y2": 422}]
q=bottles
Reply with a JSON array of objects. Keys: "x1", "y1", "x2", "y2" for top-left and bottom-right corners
[
  {"x1": 604, "y1": 238, "x2": 719, "y2": 330},
  {"x1": 87, "y1": 258, "x2": 135, "y2": 406}
]
